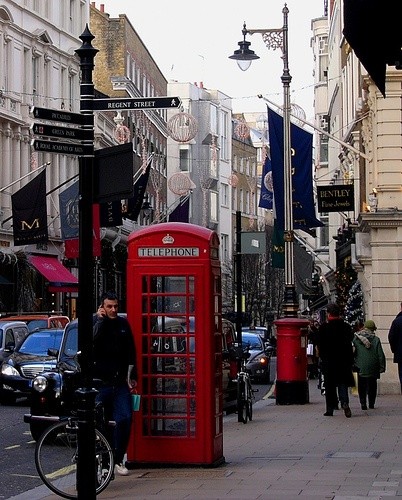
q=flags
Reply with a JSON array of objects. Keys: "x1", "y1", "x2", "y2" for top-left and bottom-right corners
[
  {"x1": 293, "y1": 243, "x2": 313, "y2": 294},
  {"x1": 271, "y1": 218, "x2": 285, "y2": 269},
  {"x1": 11, "y1": 168, "x2": 49, "y2": 246},
  {"x1": 120, "y1": 159, "x2": 152, "y2": 222},
  {"x1": 65, "y1": 203, "x2": 101, "y2": 259},
  {"x1": 258, "y1": 153, "x2": 277, "y2": 209},
  {"x1": 168, "y1": 194, "x2": 189, "y2": 223},
  {"x1": 60, "y1": 179, "x2": 79, "y2": 240},
  {"x1": 266, "y1": 105, "x2": 326, "y2": 230},
  {"x1": 99, "y1": 198, "x2": 123, "y2": 227}
]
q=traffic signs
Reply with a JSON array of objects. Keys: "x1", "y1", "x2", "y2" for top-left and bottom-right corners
[
  {"x1": 32, "y1": 124, "x2": 94, "y2": 140},
  {"x1": 78, "y1": 98, "x2": 180, "y2": 111},
  {"x1": 34, "y1": 141, "x2": 95, "y2": 156},
  {"x1": 34, "y1": 105, "x2": 95, "y2": 128}
]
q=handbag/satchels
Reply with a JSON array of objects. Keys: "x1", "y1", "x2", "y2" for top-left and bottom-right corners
[{"x1": 352, "y1": 372, "x2": 359, "y2": 394}]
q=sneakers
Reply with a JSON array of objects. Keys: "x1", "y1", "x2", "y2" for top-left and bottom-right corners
[
  {"x1": 115, "y1": 465, "x2": 128, "y2": 475},
  {"x1": 369, "y1": 404, "x2": 374, "y2": 408},
  {"x1": 362, "y1": 404, "x2": 367, "y2": 410},
  {"x1": 101, "y1": 469, "x2": 108, "y2": 476}
]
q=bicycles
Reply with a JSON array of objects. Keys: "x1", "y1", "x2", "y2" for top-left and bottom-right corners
[
  {"x1": 35, "y1": 352, "x2": 117, "y2": 499},
  {"x1": 224, "y1": 342, "x2": 262, "y2": 424}
]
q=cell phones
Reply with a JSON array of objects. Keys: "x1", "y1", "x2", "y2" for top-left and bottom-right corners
[{"x1": 100, "y1": 301, "x2": 105, "y2": 317}]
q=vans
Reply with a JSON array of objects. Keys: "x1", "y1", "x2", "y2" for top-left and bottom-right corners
[{"x1": 241, "y1": 327, "x2": 270, "y2": 346}]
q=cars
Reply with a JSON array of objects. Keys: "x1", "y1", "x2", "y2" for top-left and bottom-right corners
[
  {"x1": 0, "y1": 320, "x2": 29, "y2": 364},
  {"x1": 23, "y1": 311, "x2": 193, "y2": 442},
  {"x1": 0, "y1": 314, "x2": 70, "y2": 334},
  {"x1": 1, "y1": 327, "x2": 68, "y2": 405},
  {"x1": 234, "y1": 331, "x2": 274, "y2": 383}
]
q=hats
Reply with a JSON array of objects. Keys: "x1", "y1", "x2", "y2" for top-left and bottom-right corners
[{"x1": 365, "y1": 320, "x2": 377, "y2": 330}]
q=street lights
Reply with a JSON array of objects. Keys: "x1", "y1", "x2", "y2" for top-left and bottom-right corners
[{"x1": 227, "y1": 3, "x2": 300, "y2": 320}]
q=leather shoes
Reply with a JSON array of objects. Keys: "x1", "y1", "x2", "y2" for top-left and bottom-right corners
[
  {"x1": 343, "y1": 402, "x2": 351, "y2": 417},
  {"x1": 324, "y1": 411, "x2": 333, "y2": 415}
]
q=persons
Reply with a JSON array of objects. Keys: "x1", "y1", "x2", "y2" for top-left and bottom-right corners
[
  {"x1": 352, "y1": 320, "x2": 386, "y2": 410},
  {"x1": 388, "y1": 302, "x2": 401, "y2": 395},
  {"x1": 221, "y1": 320, "x2": 238, "y2": 379},
  {"x1": 307, "y1": 303, "x2": 356, "y2": 418},
  {"x1": 93, "y1": 290, "x2": 138, "y2": 480},
  {"x1": 353, "y1": 320, "x2": 364, "y2": 332},
  {"x1": 307, "y1": 318, "x2": 327, "y2": 379}
]
